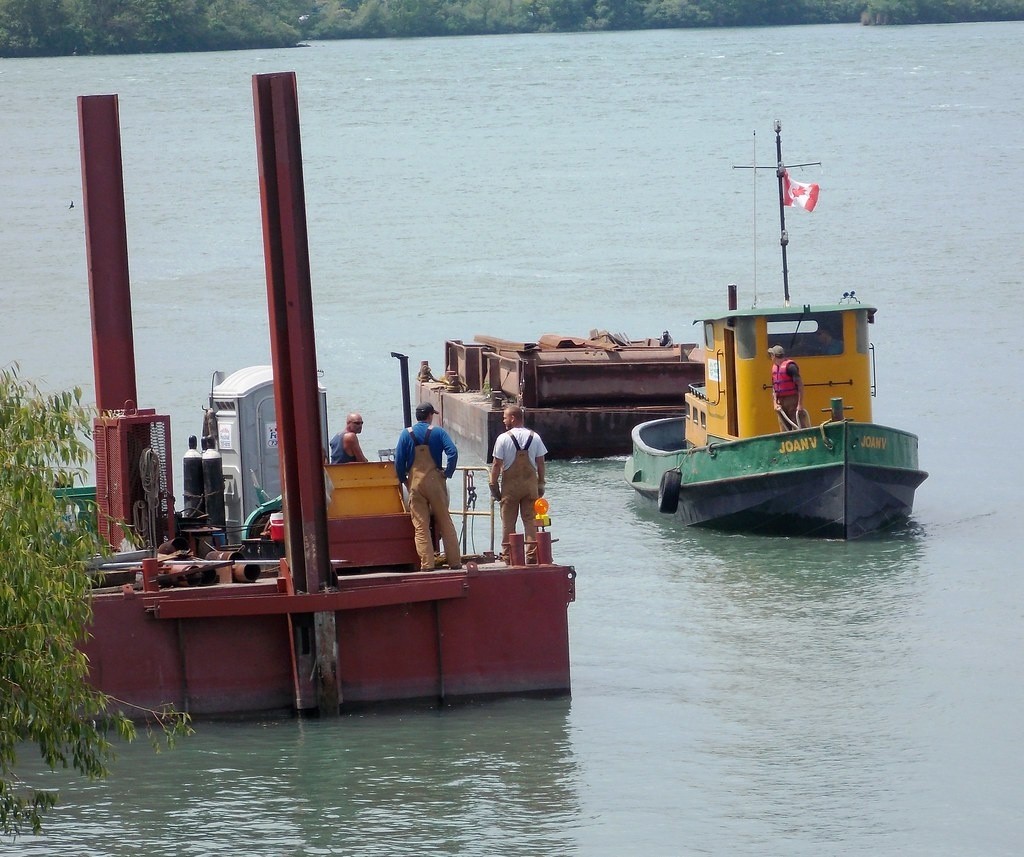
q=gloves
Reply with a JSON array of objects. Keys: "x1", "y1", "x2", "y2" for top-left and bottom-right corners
[
  {"x1": 489, "y1": 483, "x2": 501, "y2": 502},
  {"x1": 538, "y1": 481, "x2": 546, "y2": 497}
]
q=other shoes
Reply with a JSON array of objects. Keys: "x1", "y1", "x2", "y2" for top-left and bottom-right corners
[
  {"x1": 527, "y1": 558, "x2": 537, "y2": 564},
  {"x1": 504, "y1": 559, "x2": 510, "y2": 565},
  {"x1": 451, "y1": 564, "x2": 462, "y2": 569},
  {"x1": 421, "y1": 569, "x2": 434, "y2": 571}
]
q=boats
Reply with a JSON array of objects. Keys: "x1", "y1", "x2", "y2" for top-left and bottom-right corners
[
  {"x1": 416, "y1": 327, "x2": 706, "y2": 466},
  {"x1": 623, "y1": 117, "x2": 930, "y2": 546},
  {"x1": 62, "y1": 70, "x2": 579, "y2": 723}
]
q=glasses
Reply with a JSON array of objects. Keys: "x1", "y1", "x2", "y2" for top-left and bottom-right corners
[{"x1": 349, "y1": 420, "x2": 363, "y2": 425}]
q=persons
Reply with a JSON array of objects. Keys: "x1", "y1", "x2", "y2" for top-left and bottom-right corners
[
  {"x1": 767, "y1": 345, "x2": 804, "y2": 432},
  {"x1": 490, "y1": 405, "x2": 548, "y2": 564},
  {"x1": 815, "y1": 325, "x2": 844, "y2": 356},
  {"x1": 394, "y1": 400, "x2": 463, "y2": 572},
  {"x1": 330, "y1": 412, "x2": 368, "y2": 464}
]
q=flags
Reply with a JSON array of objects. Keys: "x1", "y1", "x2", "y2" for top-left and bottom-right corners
[{"x1": 782, "y1": 168, "x2": 820, "y2": 213}]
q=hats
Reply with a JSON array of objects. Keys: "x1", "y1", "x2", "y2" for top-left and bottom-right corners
[
  {"x1": 416, "y1": 402, "x2": 439, "y2": 414},
  {"x1": 768, "y1": 345, "x2": 785, "y2": 357}
]
